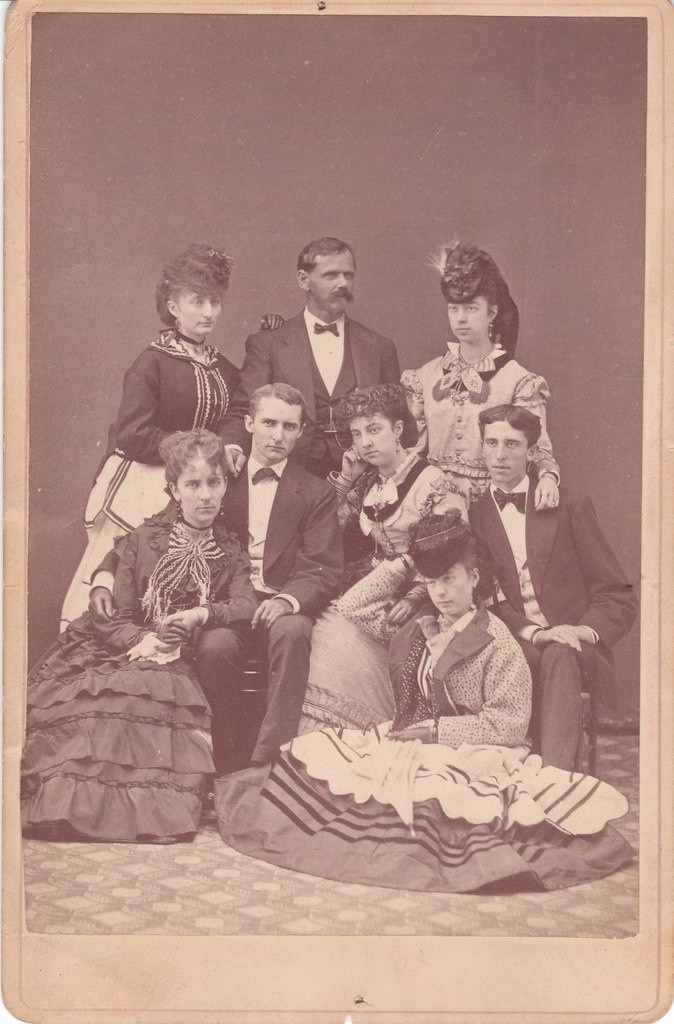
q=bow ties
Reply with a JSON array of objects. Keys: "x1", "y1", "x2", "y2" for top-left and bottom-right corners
[
  {"x1": 313, "y1": 322, "x2": 340, "y2": 337},
  {"x1": 494, "y1": 488, "x2": 526, "y2": 517},
  {"x1": 251, "y1": 467, "x2": 279, "y2": 484}
]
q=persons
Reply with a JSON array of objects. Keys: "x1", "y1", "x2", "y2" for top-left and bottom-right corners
[
  {"x1": 193, "y1": 379, "x2": 346, "y2": 771},
  {"x1": 388, "y1": 402, "x2": 639, "y2": 798},
  {"x1": 220, "y1": 232, "x2": 420, "y2": 559},
  {"x1": 215, "y1": 510, "x2": 632, "y2": 896},
  {"x1": 294, "y1": 380, "x2": 468, "y2": 759},
  {"x1": 24, "y1": 426, "x2": 258, "y2": 844},
  {"x1": 58, "y1": 239, "x2": 285, "y2": 640},
  {"x1": 399, "y1": 240, "x2": 562, "y2": 513}
]
